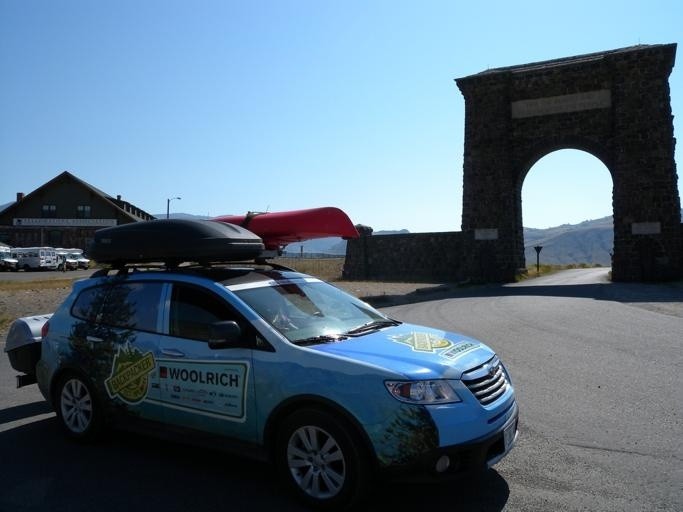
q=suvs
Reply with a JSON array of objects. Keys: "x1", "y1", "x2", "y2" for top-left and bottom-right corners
[{"x1": 36, "y1": 262, "x2": 520, "y2": 499}]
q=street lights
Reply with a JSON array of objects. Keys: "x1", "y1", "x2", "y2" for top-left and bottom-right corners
[{"x1": 167, "y1": 198, "x2": 181, "y2": 219}]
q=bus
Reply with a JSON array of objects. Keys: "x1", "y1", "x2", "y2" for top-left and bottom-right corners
[{"x1": 0, "y1": 245, "x2": 91, "y2": 271}]
